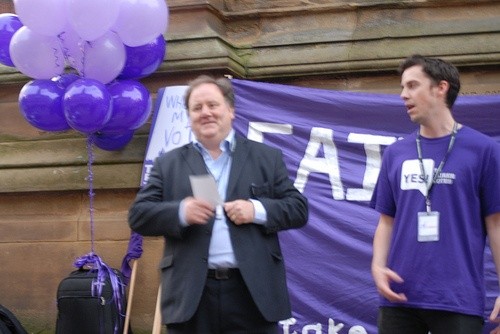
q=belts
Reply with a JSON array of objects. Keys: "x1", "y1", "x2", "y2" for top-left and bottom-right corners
[{"x1": 206, "y1": 267, "x2": 242, "y2": 279}]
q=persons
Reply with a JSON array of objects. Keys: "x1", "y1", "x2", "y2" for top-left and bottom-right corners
[
  {"x1": 128, "y1": 72, "x2": 307, "y2": 333},
  {"x1": 367, "y1": 52, "x2": 500, "y2": 334}
]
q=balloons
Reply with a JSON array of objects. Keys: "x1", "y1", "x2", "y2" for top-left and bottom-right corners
[{"x1": 0, "y1": 0, "x2": 167, "y2": 151}]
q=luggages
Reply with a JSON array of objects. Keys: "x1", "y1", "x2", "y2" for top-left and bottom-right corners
[{"x1": 53, "y1": 259, "x2": 129, "y2": 334}]
